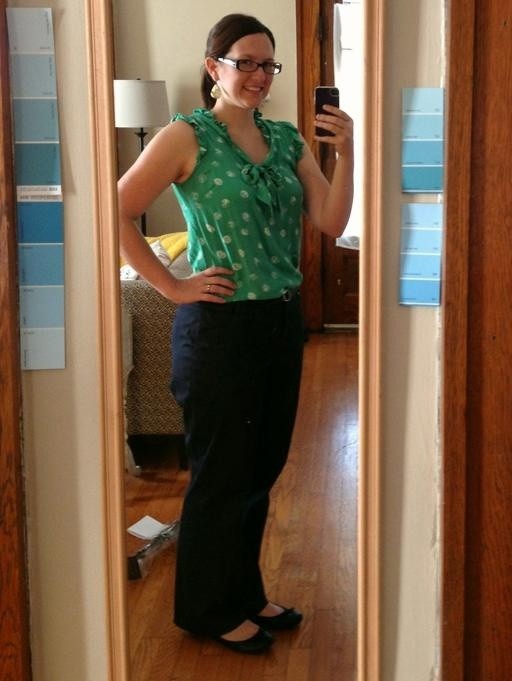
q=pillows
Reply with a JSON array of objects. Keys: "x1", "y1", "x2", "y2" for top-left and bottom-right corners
[{"x1": 118, "y1": 240, "x2": 171, "y2": 282}]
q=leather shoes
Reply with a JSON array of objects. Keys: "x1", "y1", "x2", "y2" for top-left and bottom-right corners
[
  {"x1": 253, "y1": 603, "x2": 304, "y2": 626},
  {"x1": 220, "y1": 626, "x2": 275, "y2": 653}
]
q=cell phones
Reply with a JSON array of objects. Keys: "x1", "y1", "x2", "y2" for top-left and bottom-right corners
[{"x1": 313, "y1": 86, "x2": 340, "y2": 138}]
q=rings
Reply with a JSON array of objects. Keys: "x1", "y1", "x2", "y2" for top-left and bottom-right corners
[{"x1": 207, "y1": 284, "x2": 210, "y2": 291}]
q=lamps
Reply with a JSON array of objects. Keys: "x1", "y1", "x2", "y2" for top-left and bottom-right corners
[{"x1": 113, "y1": 77, "x2": 172, "y2": 236}]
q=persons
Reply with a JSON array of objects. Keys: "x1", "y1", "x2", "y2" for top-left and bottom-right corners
[{"x1": 117, "y1": 13, "x2": 353, "y2": 656}]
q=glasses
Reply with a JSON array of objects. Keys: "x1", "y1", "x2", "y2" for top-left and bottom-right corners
[{"x1": 218, "y1": 56, "x2": 282, "y2": 75}]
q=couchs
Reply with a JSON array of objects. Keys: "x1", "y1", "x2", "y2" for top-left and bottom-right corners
[{"x1": 118, "y1": 231, "x2": 194, "y2": 470}]
